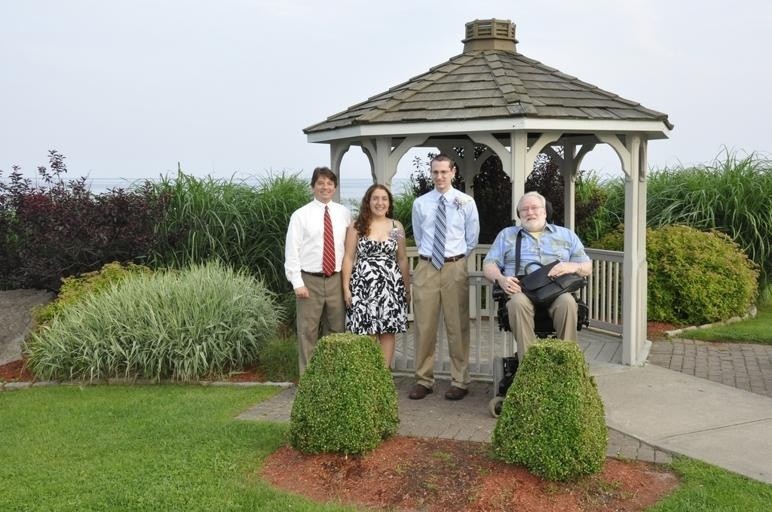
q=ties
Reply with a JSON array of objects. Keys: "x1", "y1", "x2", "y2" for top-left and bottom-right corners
[
  {"x1": 323, "y1": 205, "x2": 335, "y2": 276},
  {"x1": 431, "y1": 196, "x2": 447, "y2": 271}
]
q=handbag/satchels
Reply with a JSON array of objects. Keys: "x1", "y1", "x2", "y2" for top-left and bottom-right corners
[{"x1": 519, "y1": 260, "x2": 586, "y2": 306}]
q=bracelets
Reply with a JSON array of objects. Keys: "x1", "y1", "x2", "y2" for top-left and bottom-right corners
[{"x1": 576, "y1": 261, "x2": 582, "y2": 274}]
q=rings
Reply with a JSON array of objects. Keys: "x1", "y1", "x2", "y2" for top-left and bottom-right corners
[{"x1": 506, "y1": 285, "x2": 510, "y2": 291}]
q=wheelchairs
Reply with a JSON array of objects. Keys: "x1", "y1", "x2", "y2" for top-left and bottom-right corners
[{"x1": 487, "y1": 202, "x2": 591, "y2": 418}]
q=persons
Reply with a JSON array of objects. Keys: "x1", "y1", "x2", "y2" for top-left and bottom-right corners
[
  {"x1": 482, "y1": 192, "x2": 593, "y2": 363},
  {"x1": 340, "y1": 184, "x2": 411, "y2": 369},
  {"x1": 407, "y1": 154, "x2": 480, "y2": 399},
  {"x1": 284, "y1": 166, "x2": 352, "y2": 376}
]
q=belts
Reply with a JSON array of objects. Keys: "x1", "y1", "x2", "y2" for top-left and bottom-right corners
[
  {"x1": 309, "y1": 271, "x2": 337, "y2": 278},
  {"x1": 419, "y1": 255, "x2": 465, "y2": 263}
]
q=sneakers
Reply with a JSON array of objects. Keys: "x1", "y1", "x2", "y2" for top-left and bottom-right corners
[
  {"x1": 408, "y1": 384, "x2": 433, "y2": 399},
  {"x1": 445, "y1": 386, "x2": 468, "y2": 400}
]
